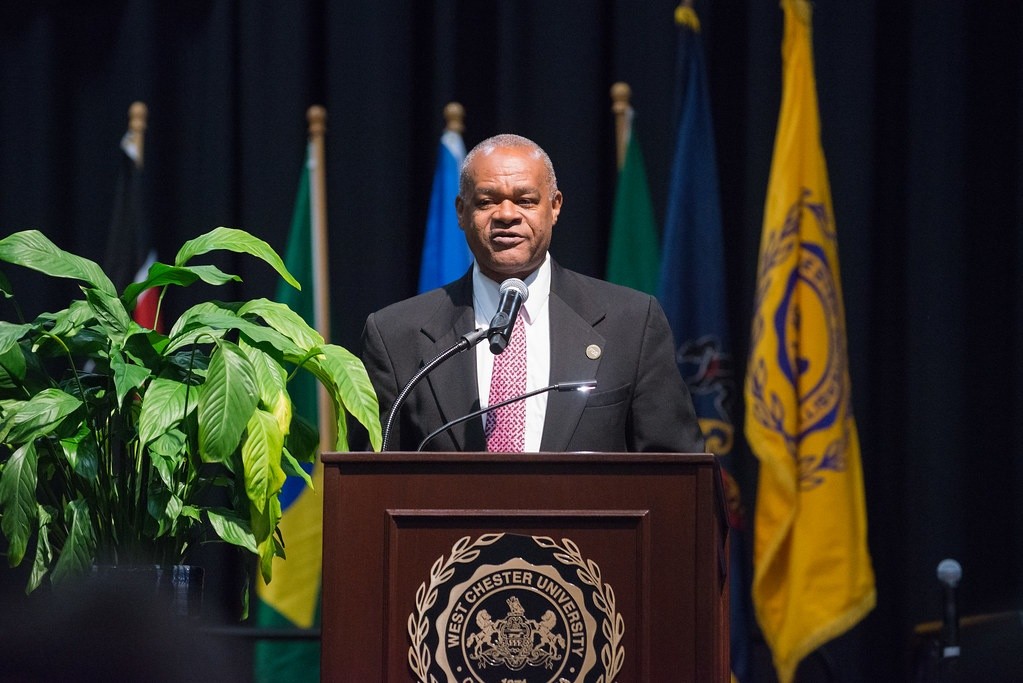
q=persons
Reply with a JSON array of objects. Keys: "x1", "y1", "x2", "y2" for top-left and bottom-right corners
[{"x1": 348, "y1": 134, "x2": 706, "y2": 454}]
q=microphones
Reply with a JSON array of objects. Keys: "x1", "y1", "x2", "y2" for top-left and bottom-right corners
[
  {"x1": 938, "y1": 559, "x2": 963, "y2": 665},
  {"x1": 494, "y1": 277, "x2": 530, "y2": 356}
]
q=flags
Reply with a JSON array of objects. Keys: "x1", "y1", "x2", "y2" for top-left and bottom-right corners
[
  {"x1": 253, "y1": 135, "x2": 346, "y2": 683},
  {"x1": 607, "y1": 106, "x2": 658, "y2": 298},
  {"x1": 743, "y1": 0, "x2": 877, "y2": 683},
  {"x1": 416, "y1": 128, "x2": 467, "y2": 294},
  {"x1": 83, "y1": 131, "x2": 164, "y2": 379},
  {"x1": 655, "y1": 7, "x2": 750, "y2": 683}
]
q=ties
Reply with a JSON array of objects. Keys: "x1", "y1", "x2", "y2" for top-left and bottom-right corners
[{"x1": 486, "y1": 305, "x2": 527, "y2": 453}]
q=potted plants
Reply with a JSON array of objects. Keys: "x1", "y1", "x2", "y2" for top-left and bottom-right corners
[{"x1": 0, "y1": 226, "x2": 384, "y2": 631}]
